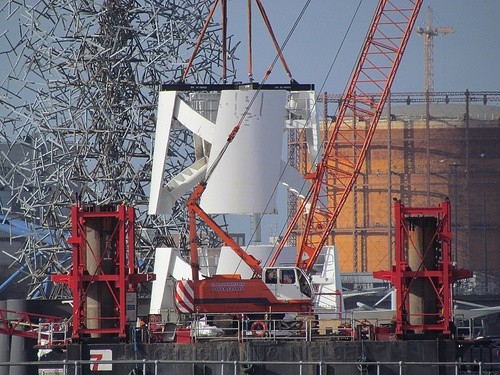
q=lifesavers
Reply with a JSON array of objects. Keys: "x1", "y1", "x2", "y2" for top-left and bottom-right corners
[{"x1": 250, "y1": 321, "x2": 266, "y2": 337}]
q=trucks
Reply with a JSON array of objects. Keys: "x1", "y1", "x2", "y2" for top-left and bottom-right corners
[{"x1": 174, "y1": 265, "x2": 316, "y2": 337}]
